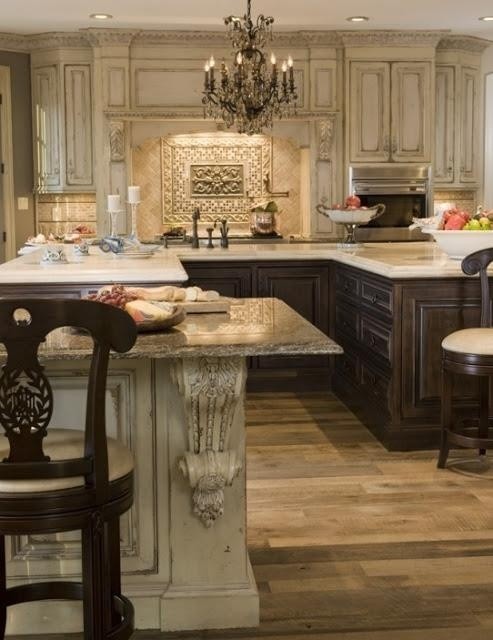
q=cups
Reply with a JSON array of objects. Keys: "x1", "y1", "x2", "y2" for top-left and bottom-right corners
[
  {"x1": 72, "y1": 242, "x2": 89, "y2": 255},
  {"x1": 42, "y1": 244, "x2": 69, "y2": 264}
]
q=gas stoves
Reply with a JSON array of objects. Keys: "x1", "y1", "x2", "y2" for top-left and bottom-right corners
[{"x1": 156, "y1": 231, "x2": 283, "y2": 240}]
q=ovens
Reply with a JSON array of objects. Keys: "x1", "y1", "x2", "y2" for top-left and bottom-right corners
[{"x1": 349, "y1": 162, "x2": 431, "y2": 242}]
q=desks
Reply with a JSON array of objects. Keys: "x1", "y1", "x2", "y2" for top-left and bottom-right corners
[{"x1": 0, "y1": 293, "x2": 343, "y2": 634}]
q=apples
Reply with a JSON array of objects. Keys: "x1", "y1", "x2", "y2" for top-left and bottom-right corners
[
  {"x1": 332, "y1": 194, "x2": 368, "y2": 210},
  {"x1": 439, "y1": 208, "x2": 493, "y2": 230}
]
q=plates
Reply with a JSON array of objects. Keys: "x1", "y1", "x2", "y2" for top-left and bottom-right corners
[{"x1": 65, "y1": 233, "x2": 95, "y2": 237}]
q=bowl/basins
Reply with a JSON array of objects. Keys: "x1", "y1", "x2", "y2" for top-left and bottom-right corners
[
  {"x1": 250, "y1": 211, "x2": 276, "y2": 234},
  {"x1": 419, "y1": 224, "x2": 492, "y2": 259}
]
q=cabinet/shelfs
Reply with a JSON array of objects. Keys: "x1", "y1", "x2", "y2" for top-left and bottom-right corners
[
  {"x1": 396, "y1": 281, "x2": 493, "y2": 426},
  {"x1": 332, "y1": 264, "x2": 390, "y2": 432},
  {"x1": 179, "y1": 261, "x2": 258, "y2": 375},
  {"x1": 259, "y1": 262, "x2": 332, "y2": 372},
  {"x1": 346, "y1": 55, "x2": 432, "y2": 167},
  {"x1": 436, "y1": 65, "x2": 477, "y2": 188},
  {"x1": 30, "y1": 51, "x2": 94, "y2": 192}
]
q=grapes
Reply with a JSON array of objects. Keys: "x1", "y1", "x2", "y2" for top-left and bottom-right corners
[{"x1": 86, "y1": 285, "x2": 145, "y2": 310}]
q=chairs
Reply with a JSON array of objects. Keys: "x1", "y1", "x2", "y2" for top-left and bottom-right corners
[
  {"x1": 433, "y1": 249, "x2": 493, "y2": 472},
  {"x1": 1, "y1": 293, "x2": 140, "y2": 639}
]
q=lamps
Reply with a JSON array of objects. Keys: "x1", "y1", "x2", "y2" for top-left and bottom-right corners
[{"x1": 197, "y1": 1, "x2": 300, "y2": 133}]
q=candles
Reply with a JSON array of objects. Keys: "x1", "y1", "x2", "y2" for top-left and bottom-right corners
[
  {"x1": 129, "y1": 187, "x2": 139, "y2": 204},
  {"x1": 107, "y1": 194, "x2": 121, "y2": 209}
]
